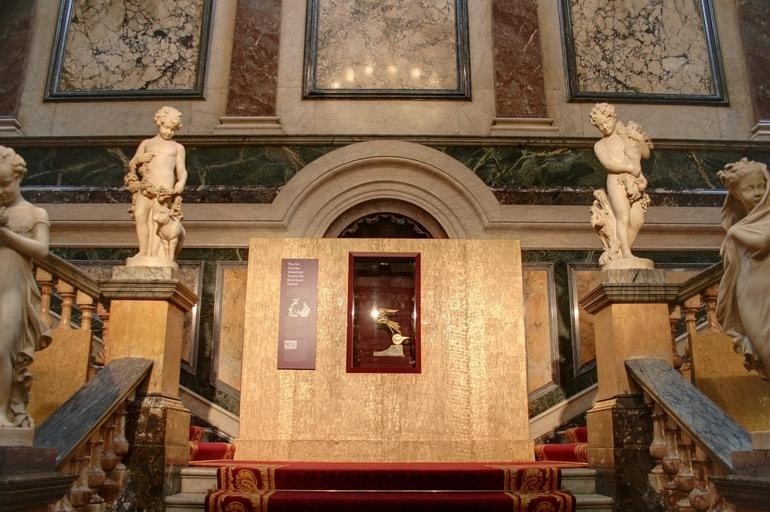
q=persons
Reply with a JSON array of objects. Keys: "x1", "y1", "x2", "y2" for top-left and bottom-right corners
[
  {"x1": 125, "y1": 105, "x2": 190, "y2": 260},
  {"x1": 589, "y1": 102, "x2": 651, "y2": 259},
  {"x1": 0, "y1": 143, "x2": 49, "y2": 430},
  {"x1": 718, "y1": 158, "x2": 770, "y2": 387}
]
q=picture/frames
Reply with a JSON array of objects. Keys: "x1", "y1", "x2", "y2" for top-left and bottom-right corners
[
  {"x1": 557, "y1": 0, "x2": 730, "y2": 106},
  {"x1": 302, "y1": 0, "x2": 471, "y2": 100},
  {"x1": 44, "y1": 0, "x2": 216, "y2": 102}
]
